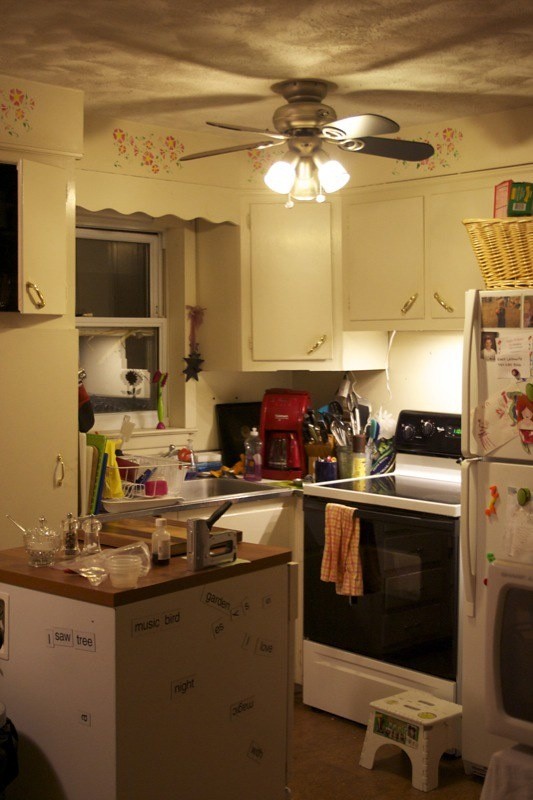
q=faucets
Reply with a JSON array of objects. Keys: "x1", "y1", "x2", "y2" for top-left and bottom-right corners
[{"x1": 163, "y1": 444, "x2": 192, "y2": 468}]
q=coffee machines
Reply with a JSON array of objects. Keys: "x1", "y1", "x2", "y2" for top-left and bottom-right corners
[{"x1": 259, "y1": 387, "x2": 316, "y2": 480}]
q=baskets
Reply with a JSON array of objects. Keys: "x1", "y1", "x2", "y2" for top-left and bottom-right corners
[{"x1": 462, "y1": 216, "x2": 533, "y2": 290}]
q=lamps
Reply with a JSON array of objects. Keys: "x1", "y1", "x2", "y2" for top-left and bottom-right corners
[{"x1": 264, "y1": 138, "x2": 350, "y2": 208}]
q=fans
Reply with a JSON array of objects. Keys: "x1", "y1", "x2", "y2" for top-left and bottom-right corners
[{"x1": 180, "y1": 79, "x2": 435, "y2": 162}]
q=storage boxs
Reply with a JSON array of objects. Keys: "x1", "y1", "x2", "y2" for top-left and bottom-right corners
[{"x1": 494, "y1": 178, "x2": 533, "y2": 218}]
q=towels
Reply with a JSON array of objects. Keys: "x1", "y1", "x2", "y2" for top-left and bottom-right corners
[{"x1": 319, "y1": 502, "x2": 365, "y2": 598}]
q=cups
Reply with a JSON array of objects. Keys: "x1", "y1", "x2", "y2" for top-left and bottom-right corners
[
  {"x1": 107, "y1": 555, "x2": 142, "y2": 589},
  {"x1": 338, "y1": 446, "x2": 351, "y2": 478}
]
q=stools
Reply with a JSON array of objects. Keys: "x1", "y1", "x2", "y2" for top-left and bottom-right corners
[{"x1": 359, "y1": 689, "x2": 462, "y2": 792}]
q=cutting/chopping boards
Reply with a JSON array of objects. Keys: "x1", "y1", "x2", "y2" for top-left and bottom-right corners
[{"x1": 215, "y1": 401, "x2": 261, "y2": 475}]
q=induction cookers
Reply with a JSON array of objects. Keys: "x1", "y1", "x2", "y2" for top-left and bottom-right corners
[{"x1": 301, "y1": 410, "x2": 465, "y2": 517}]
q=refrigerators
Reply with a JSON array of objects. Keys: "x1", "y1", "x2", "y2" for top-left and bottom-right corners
[{"x1": 457, "y1": 289, "x2": 533, "y2": 769}]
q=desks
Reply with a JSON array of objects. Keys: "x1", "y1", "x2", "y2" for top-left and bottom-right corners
[{"x1": 0, "y1": 519, "x2": 299, "y2": 800}]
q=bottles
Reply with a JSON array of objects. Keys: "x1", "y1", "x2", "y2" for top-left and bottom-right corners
[
  {"x1": 21, "y1": 515, "x2": 60, "y2": 566},
  {"x1": 244, "y1": 427, "x2": 261, "y2": 482},
  {"x1": 351, "y1": 435, "x2": 370, "y2": 478},
  {"x1": 61, "y1": 513, "x2": 80, "y2": 559},
  {"x1": 82, "y1": 514, "x2": 102, "y2": 555},
  {"x1": 151, "y1": 518, "x2": 171, "y2": 569}
]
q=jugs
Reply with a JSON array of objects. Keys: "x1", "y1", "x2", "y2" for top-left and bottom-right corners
[{"x1": 266, "y1": 433, "x2": 301, "y2": 469}]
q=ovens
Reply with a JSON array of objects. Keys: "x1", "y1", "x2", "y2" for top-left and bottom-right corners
[{"x1": 302, "y1": 494, "x2": 459, "y2": 726}]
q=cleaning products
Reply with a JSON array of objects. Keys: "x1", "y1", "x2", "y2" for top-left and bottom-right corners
[
  {"x1": 244, "y1": 427, "x2": 263, "y2": 481},
  {"x1": 186, "y1": 439, "x2": 198, "y2": 474}
]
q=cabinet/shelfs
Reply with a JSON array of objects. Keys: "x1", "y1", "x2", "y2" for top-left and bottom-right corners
[
  {"x1": 0, "y1": 151, "x2": 76, "y2": 316},
  {"x1": 0, "y1": 326, "x2": 78, "y2": 551},
  {"x1": 195, "y1": 193, "x2": 389, "y2": 371},
  {"x1": 341, "y1": 164, "x2": 533, "y2": 331}
]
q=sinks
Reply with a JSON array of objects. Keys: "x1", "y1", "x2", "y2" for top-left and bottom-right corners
[{"x1": 178, "y1": 477, "x2": 295, "y2": 502}]
